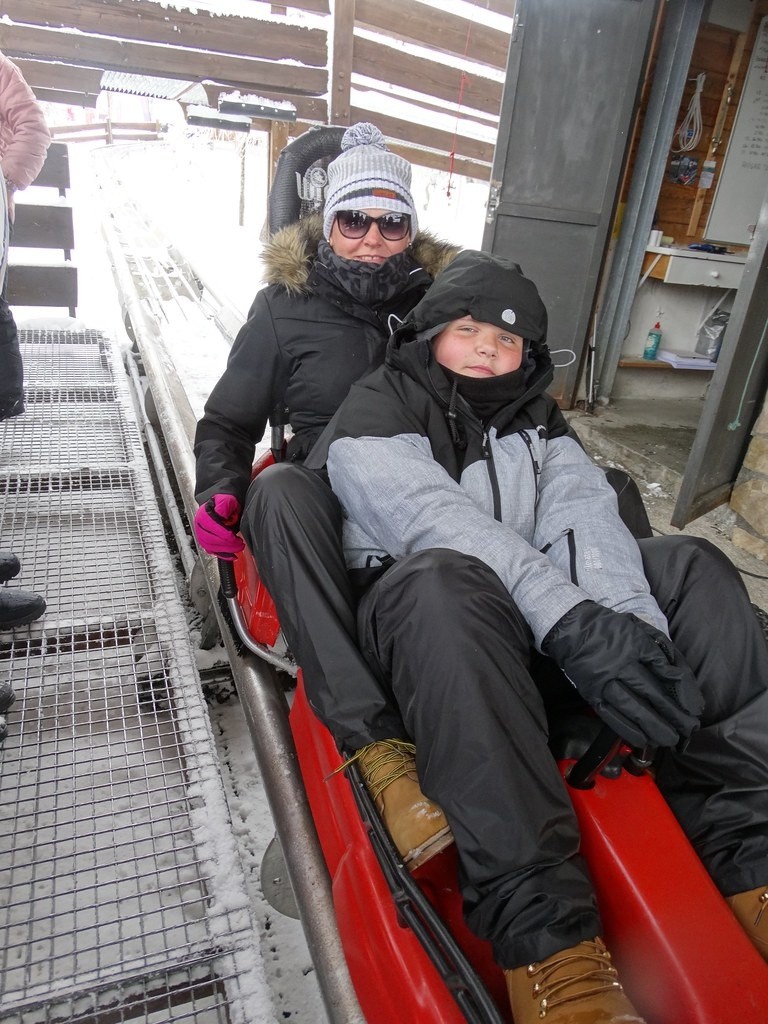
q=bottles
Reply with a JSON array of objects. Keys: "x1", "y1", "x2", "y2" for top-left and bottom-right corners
[{"x1": 643, "y1": 324, "x2": 663, "y2": 360}]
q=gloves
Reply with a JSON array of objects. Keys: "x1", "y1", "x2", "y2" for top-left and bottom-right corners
[
  {"x1": 544, "y1": 601, "x2": 704, "y2": 777},
  {"x1": 195, "y1": 493, "x2": 245, "y2": 561}
]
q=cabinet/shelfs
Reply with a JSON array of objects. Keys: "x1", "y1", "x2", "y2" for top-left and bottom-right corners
[{"x1": 616, "y1": 243, "x2": 747, "y2": 368}]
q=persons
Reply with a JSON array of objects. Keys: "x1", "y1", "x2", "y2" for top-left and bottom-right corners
[
  {"x1": 324, "y1": 250, "x2": 768, "y2": 1024},
  {"x1": 194, "y1": 122, "x2": 656, "y2": 873},
  {"x1": 0, "y1": 53, "x2": 54, "y2": 743}
]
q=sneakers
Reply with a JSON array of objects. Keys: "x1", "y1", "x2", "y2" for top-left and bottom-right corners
[
  {"x1": 507, "y1": 935, "x2": 645, "y2": 1024},
  {"x1": 728, "y1": 883, "x2": 767, "y2": 959},
  {"x1": 322, "y1": 739, "x2": 453, "y2": 871}
]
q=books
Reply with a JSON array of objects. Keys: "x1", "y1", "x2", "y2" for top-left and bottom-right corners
[{"x1": 656, "y1": 346, "x2": 717, "y2": 370}]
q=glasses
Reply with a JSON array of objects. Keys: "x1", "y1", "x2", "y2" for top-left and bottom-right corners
[{"x1": 336, "y1": 210, "x2": 411, "y2": 241}]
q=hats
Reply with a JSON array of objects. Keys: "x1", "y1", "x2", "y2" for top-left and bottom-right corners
[{"x1": 323, "y1": 122, "x2": 418, "y2": 244}]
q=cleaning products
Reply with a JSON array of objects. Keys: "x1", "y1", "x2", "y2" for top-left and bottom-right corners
[{"x1": 643, "y1": 322, "x2": 663, "y2": 360}]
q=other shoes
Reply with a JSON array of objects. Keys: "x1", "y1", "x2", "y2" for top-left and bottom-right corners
[
  {"x1": 0, "y1": 552, "x2": 20, "y2": 584},
  {"x1": 0, "y1": 587, "x2": 46, "y2": 631}
]
